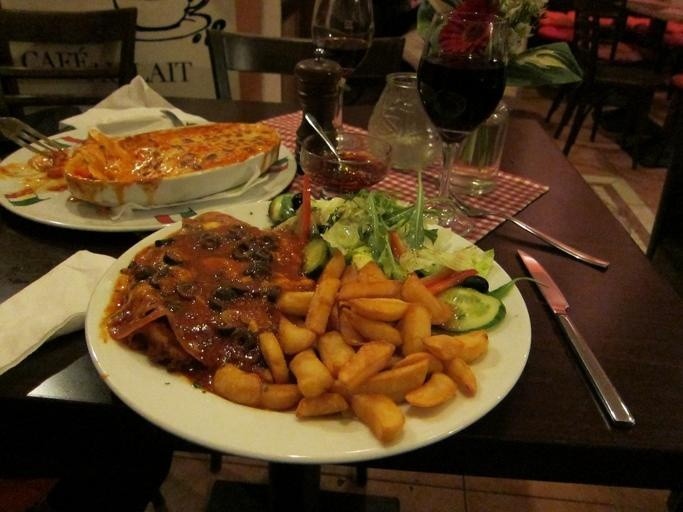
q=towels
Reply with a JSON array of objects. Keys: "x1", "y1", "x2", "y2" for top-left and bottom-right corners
[{"x1": 285, "y1": 122, "x2": 550, "y2": 245}]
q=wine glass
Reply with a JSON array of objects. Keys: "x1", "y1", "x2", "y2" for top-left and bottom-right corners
[
  {"x1": 413, "y1": 10, "x2": 511, "y2": 238},
  {"x1": 309, "y1": 0, "x2": 376, "y2": 134}
]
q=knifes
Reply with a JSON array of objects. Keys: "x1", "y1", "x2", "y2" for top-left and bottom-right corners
[{"x1": 515, "y1": 245, "x2": 639, "y2": 428}]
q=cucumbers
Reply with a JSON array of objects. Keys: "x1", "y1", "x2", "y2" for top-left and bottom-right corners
[{"x1": 434, "y1": 284, "x2": 507, "y2": 333}]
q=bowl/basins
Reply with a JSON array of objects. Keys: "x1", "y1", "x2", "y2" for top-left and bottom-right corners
[{"x1": 299, "y1": 130, "x2": 396, "y2": 199}]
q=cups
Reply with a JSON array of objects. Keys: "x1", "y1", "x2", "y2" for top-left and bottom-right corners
[{"x1": 437, "y1": 99, "x2": 510, "y2": 197}]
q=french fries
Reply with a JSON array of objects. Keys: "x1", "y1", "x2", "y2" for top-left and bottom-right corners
[{"x1": 213, "y1": 250, "x2": 488, "y2": 441}]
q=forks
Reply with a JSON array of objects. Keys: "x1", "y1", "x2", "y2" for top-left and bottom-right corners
[
  {"x1": 0, "y1": 114, "x2": 62, "y2": 164},
  {"x1": 432, "y1": 176, "x2": 612, "y2": 271}
]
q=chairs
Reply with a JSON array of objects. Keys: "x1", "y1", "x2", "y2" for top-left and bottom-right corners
[
  {"x1": 0, "y1": 8, "x2": 138, "y2": 116},
  {"x1": 543, "y1": 0, "x2": 664, "y2": 171},
  {"x1": 204, "y1": 26, "x2": 406, "y2": 109}
]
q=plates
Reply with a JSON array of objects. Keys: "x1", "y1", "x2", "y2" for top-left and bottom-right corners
[
  {"x1": 82, "y1": 199, "x2": 533, "y2": 467},
  {"x1": 60, "y1": 133, "x2": 283, "y2": 213},
  {"x1": 0, "y1": 128, "x2": 300, "y2": 233}
]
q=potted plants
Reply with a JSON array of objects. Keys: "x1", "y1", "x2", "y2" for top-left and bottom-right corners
[{"x1": 416, "y1": 0, "x2": 586, "y2": 197}]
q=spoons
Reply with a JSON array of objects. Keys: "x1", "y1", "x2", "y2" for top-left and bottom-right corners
[{"x1": 300, "y1": 113, "x2": 377, "y2": 180}]
